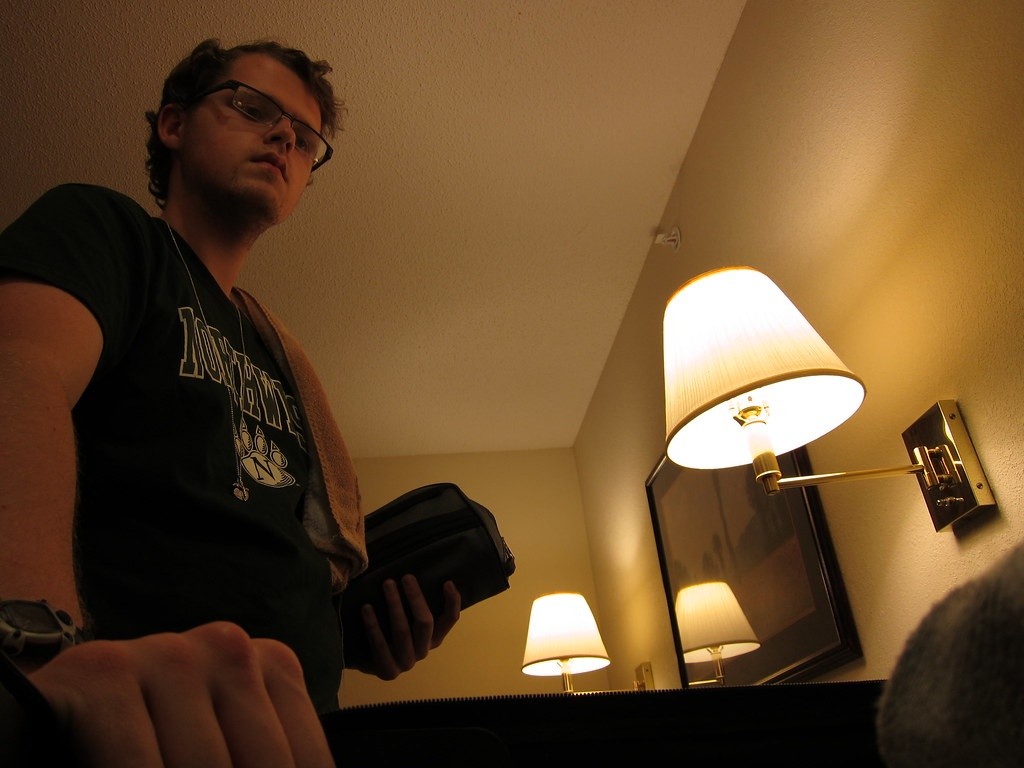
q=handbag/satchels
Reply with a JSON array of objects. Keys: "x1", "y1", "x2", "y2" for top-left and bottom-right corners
[{"x1": 345, "y1": 483, "x2": 514, "y2": 667}]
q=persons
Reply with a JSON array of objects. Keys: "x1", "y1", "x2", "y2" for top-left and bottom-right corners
[{"x1": 0, "y1": 38, "x2": 460, "y2": 768}]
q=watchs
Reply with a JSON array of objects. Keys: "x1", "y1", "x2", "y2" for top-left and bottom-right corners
[{"x1": 0, "y1": 599, "x2": 85, "y2": 665}]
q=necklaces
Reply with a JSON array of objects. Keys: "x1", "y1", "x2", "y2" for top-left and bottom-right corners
[{"x1": 165, "y1": 219, "x2": 249, "y2": 497}]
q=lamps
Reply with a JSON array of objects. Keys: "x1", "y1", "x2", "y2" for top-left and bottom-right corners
[
  {"x1": 675, "y1": 582, "x2": 760, "y2": 685},
  {"x1": 521, "y1": 593, "x2": 656, "y2": 693},
  {"x1": 661, "y1": 265, "x2": 998, "y2": 533}
]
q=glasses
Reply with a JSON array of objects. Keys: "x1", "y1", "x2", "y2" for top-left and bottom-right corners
[{"x1": 184, "y1": 78, "x2": 334, "y2": 173}]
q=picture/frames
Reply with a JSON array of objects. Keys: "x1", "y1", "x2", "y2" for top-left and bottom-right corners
[{"x1": 645, "y1": 435, "x2": 862, "y2": 688}]
